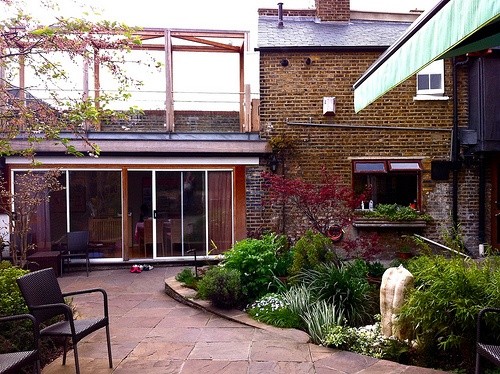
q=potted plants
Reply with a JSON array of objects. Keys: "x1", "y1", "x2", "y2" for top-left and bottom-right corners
[{"x1": 399, "y1": 246, "x2": 413, "y2": 259}]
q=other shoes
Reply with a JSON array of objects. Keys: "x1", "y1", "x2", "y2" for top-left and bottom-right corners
[{"x1": 130, "y1": 264, "x2": 154, "y2": 273}]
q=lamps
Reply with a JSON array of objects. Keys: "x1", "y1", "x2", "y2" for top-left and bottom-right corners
[
  {"x1": 269, "y1": 155, "x2": 280, "y2": 174},
  {"x1": 322, "y1": 96, "x2": 337, "y2": 117}
]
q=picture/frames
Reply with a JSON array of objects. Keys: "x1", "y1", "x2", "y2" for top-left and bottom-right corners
[{"x1": 54, "y1": 178, "x2": 87, "y2": 213}]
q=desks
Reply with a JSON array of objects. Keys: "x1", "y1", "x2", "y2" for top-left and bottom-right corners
[
  {"x1": 135, "y1": 222, "x2": 171, "y2": 251},
  {"x1": 26, "y1": 250, "x2": 61, "y2": 277}
]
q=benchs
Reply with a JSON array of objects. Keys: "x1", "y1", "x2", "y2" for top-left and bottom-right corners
[{"x1": 87, "y1": 217, "x2": 122, "y2": 253}]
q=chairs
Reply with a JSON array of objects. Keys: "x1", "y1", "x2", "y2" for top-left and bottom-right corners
[
  {"x1": 0, "y1": 313, "x2": 41, "y2": 374},
  {"x1": 53, "y1": 231, "x2": 93, "y2": 278},
  {"x1": 140, "y1": 218, "x2": 166, "y2": 258},
  {"x1": 15, "y1": 267, "x2": 113, "y2": 374},
  {"x1": 473, "y1": 307, "x2": 500, "y2": 374},
  {"x1": 165, "y1": 219, "x2": 193, "y2": 256}
]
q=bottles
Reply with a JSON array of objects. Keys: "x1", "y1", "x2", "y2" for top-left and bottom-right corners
[
  {"x1": 368, "y1": 200, "x2": 373, "y2": 210},
  {"x1": 361, "y1": 201, "x2": 365, "y2": 210}
]
